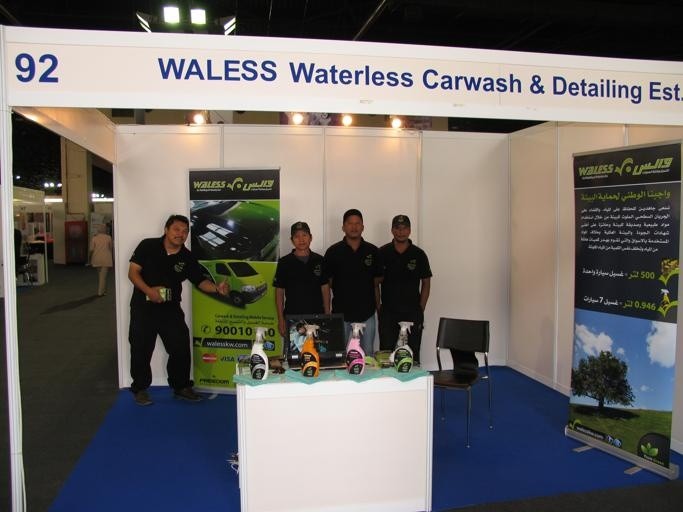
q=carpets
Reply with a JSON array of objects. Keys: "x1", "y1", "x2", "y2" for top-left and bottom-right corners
[{"x1": 48, "y1": 365, "x2": 682, "y2": 512}]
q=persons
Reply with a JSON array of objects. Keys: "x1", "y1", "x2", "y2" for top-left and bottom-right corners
[
  {"x1": 271, "y1": 221, "x2": 323, "y2": 345},
  {"x1": 294, "y1": 323, "x2": 307, "y2": 352},
  {"x1": 128, "y1": 214, "x2": 230, "y2": 406},
  {"x1": 319, "y1": 208, "x2": 379, "y2": 355},
  {"x1": 87, "y1": 224, "x2": 113, "y2": 297},
  {"x1": 373, "y1": 215, "x2": 433, "y2": 367}
]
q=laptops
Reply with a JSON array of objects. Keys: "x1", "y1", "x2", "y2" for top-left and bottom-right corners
[{"x1": 285, "y1": 313, "x2": 348, "y2": 371}]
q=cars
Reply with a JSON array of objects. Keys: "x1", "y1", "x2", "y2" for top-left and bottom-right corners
[{"x1": 189, "y1": 201, "x2": 280, "y2": 262}]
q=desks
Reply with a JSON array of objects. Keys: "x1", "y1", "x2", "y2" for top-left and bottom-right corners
[{"x1": 235, "y1": 362, "x2": 434, "y2": 512}]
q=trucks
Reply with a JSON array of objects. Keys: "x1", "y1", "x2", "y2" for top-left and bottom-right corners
[{"x1": 195, "y1": 261, "x2": 268, "y2": 307}]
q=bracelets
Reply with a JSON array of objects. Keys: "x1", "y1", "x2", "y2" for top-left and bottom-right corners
[{"x1": 216, "y1": 286, "x2": 218, "y2": 292}]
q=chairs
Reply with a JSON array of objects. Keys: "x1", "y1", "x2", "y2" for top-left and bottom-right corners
[
  {"x1": 429, "y1": 316, "x2": 493, "y2": 449},
  {"x1": 14, "y1": 228, "x2": 33, "y2": 289}
]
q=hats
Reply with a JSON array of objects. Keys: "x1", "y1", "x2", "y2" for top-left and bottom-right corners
[
  {"x1": 290, "y1": 222, "x2": 311, "y2": 237},
  {"x1": 392, "y1": 215, "x2": 411, "y2": 228}
]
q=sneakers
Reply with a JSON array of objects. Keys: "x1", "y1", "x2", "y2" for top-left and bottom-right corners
[
  {"x1": 135, "y1": 392, "x2": 152, "y2": 407},
  {"x1": 175, "y1": 389, "x2": 203, "y2": 403}
]
